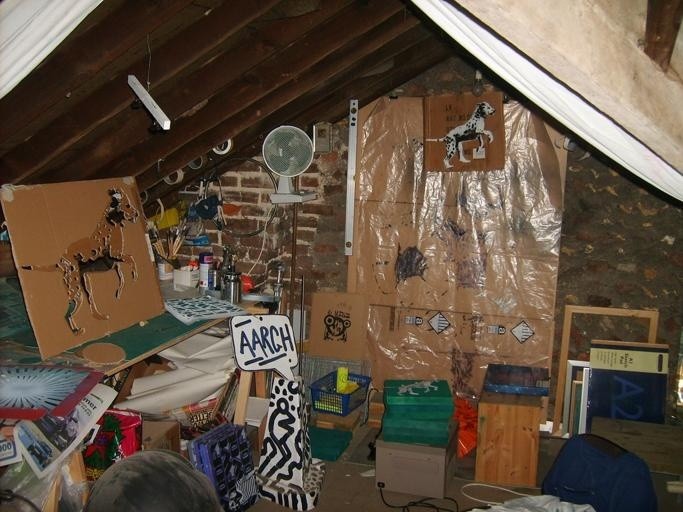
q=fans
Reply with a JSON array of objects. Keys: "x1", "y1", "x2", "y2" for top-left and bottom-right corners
[{"x1": 262, "y1": 122, "x2": 319, "y2": 205}]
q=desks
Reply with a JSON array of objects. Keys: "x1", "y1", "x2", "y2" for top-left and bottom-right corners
[{"x1": 1, "y1": 272, "x2": 268, "y2": 511}]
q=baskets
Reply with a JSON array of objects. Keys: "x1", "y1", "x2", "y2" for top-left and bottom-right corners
[{"x1": 309, "y1": 370, "x2": 372, "y2": 416}]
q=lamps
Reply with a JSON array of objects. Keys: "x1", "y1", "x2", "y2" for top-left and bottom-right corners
[{"x1": 125, "y1": 30, "x2": 171, "y2": 132}]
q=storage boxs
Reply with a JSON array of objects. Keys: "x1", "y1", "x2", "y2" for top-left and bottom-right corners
[{"x1": 372, "y1": 374, "x2": 461, "y2": 501}]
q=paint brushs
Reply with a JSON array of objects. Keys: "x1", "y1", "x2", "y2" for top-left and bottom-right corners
[{"x1": 143, "y1": 216, "x2": 192, "y2": 260}]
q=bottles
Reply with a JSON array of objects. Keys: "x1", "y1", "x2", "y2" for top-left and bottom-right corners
[
  {"x1": 220, "y1": 246, "x2": 235, "y2": 280},
  {"x1": 219, "y1": 273, "x2": 239, "y2": 304},
  {"x1": 198, "y1": 252, "x2": 214, "y2": 295}
]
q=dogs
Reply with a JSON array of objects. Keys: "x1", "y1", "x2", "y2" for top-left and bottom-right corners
[{"x1": 425, "y1": 101, "x2": 496, "y2": 169}]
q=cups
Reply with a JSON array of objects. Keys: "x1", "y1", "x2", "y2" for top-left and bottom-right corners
[
  {"x1": 157, "y1": 257, "x2": 175, "y2": 281},
  {"x1": 336, "y1": 366, "x2": 347, "y2": 394}
]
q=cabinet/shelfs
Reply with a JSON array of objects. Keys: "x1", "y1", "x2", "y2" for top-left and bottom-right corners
[{"x1": 475, "y1": 390, "x2": 545, "y2": 493}]
